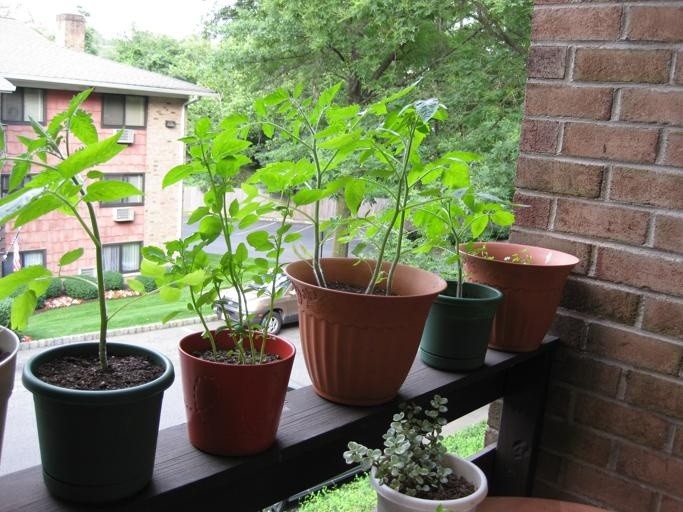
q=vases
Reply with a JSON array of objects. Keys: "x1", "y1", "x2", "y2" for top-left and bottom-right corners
[{"x1": 0, "y1": 323, "x2": 21, "y2": 465}]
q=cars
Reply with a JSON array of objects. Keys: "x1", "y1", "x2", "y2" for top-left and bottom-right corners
[{"x1": 208, "y1": 264, "x2": 299, "y2": 336}]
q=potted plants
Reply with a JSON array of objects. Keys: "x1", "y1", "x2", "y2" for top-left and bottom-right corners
[
  {"x1": 0, "y1": 88, "x2": 207, "y2": 504},
  {"x1": 344, "y1": 394, "x2": 489, "y2": 511},
  {"x1": 403, "y1": 150, "x2": 533, "y2": 372},
  {"x1": 455, "y1": 240, "x2": 580, "y2": 353},
  {"x1": 245, "y1": 74, "x2": 454, "y2": 407},
  {"x1": 146, "y1": 98, "x2": 326, "y2": 456}
]
